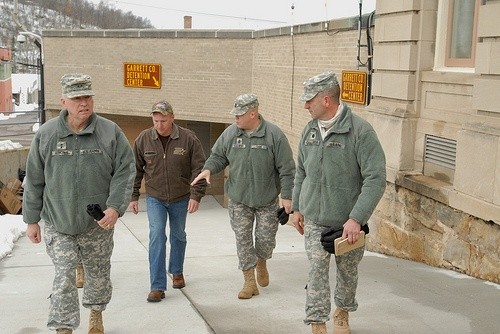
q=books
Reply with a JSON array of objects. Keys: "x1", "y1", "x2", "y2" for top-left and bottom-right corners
[{"x1": 334, "y1": 231, "x2": 364, "y2": 256}]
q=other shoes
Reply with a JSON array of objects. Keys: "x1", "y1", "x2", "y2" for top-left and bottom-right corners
[
  {"x1": 146, "y1": 291, "x2": 166, "y2": 302},
  {"x1": 172, "y1": 273, "x2": 186, "y2": 289}
]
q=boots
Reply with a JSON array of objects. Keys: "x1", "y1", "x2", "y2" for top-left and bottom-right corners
[
  {"x1": 238, "y1": 268, "x2": 259, "y2": 298},
  {"x1": 75, "y1": 267, "x2": 85, "y2": 288},
  {"x1": 55, "y1": 328, "x2": 72, "y2": 334},
  {"x1": 87, "y1": 309, "x2": 104, "y2": 334},
  {"x1": 333, "y1": 307, "x2": 351, "y2": 334},
  {"x1": 312, "y1": 324, "x2": 327, "y2": 334},
  {"x1": 256, "y1": 256, "x2": 269, "y2": 287}
]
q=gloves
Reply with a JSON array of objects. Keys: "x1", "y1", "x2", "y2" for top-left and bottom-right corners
[
  {"x1": 276, "y1": 207, "x2": 294, "y2": 226},
  {"x1": 86, "y1": 203, "x2": 106, "y2": 222},
  {"x1": 321, "y1": 223, "x2": 370, "y2": 254}
]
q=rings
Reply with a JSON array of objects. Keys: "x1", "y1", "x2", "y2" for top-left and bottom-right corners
[{"x1": 107, "y1": 224, "x2": 111, "y2": 228}]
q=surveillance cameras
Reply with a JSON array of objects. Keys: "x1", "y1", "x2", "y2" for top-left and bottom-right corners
[{"x1": 17, "y1": 36, "x2": 25, "y2": 43}]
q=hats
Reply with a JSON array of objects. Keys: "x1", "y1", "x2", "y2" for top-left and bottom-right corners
[
  {"x1": 150, "y1": 100, "x2": 173, "y2": 117},
  {"x1": 60, "y1": 73, "x2": 95, "y2": 99},
  {"x1": 229, "y1": 94, "x2": 259, "y2": 116},
  {"x1": 298, "y1": 72, "x2": 340, "y2": 102}
]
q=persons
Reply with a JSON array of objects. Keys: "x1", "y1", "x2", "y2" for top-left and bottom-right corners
[
  {"x1": 129, "y1": 101, "x2": 207, "y2": 301},
  {"x1": 291, "y1": 71, "x2": 386, "y2": 334},
  {"x1": 191, "y1": 94, "x2": 296, "y2": 298},
  {"x1": 22, "y1": 73, "x2": 136, "y2": 333}
]
